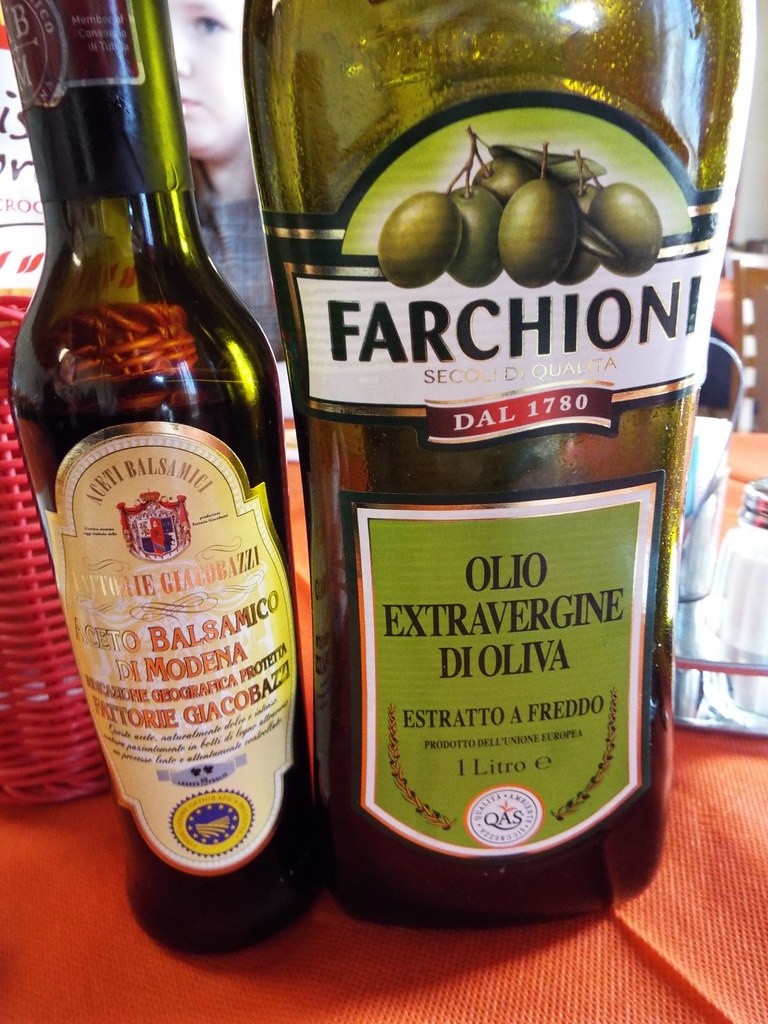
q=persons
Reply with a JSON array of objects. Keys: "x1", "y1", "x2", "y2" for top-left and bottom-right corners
[{"x1": 167, "y1": 0, "x2": 286, "y2": 363}]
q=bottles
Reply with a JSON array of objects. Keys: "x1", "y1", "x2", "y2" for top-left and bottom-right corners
[
  {"x1": 242, "y1": 1, "x2": 742, "y2": 928},
  {"x1": 722, "y1": 477, "x2": 767, "y2": 723},
  {"x1": 1, "y1": 0, "x2": 327, "y2": 955}
]
